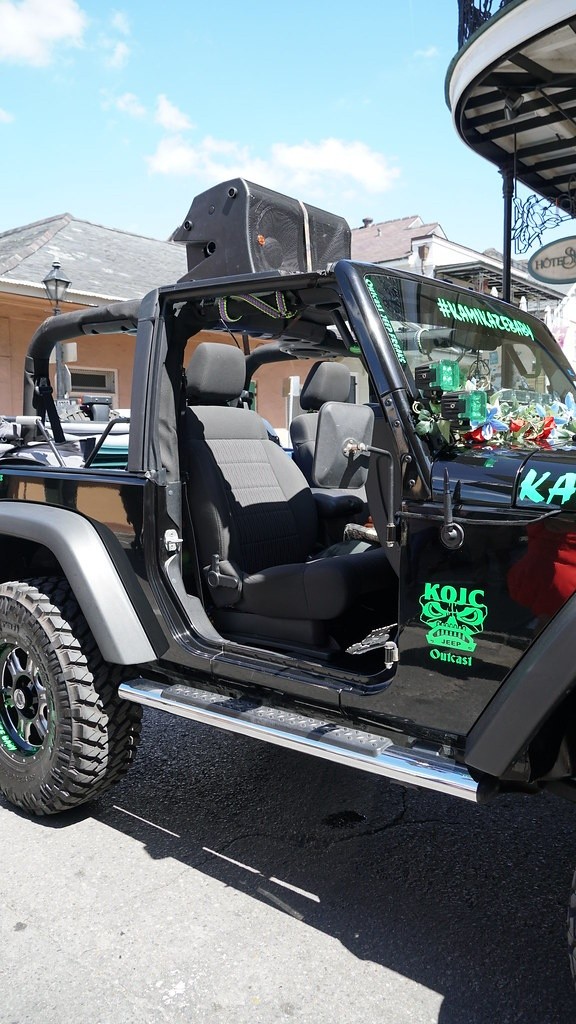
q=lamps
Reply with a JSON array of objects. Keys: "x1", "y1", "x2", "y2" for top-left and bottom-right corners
[{"x1": 417, "y1": 244, "x2": 429, "y2": 275}]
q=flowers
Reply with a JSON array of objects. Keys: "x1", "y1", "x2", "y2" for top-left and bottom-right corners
[{"x1": 415, "y1": 385, "x2": 576, "y2": 453}]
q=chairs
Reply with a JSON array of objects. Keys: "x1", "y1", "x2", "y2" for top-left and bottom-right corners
[
  {"x1": 291, "y1": 361, "x2": 351, "y2": 488},
  {"x1": 178, "y1": 342, "x2": 389, "y2": 646}
]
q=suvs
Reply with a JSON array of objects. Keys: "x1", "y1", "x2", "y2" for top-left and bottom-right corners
[{"x1": 0, "y1": 179, "x2": 576, "y2": 1003}]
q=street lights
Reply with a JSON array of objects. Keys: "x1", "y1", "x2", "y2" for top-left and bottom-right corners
[{"x1": 43, "y1": 258, "x2": 70, "y2": 400}]
